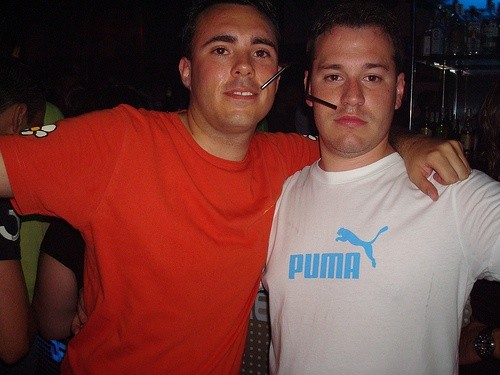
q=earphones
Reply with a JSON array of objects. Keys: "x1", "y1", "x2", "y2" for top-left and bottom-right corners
[{"x1": 305, "y1": 71, "x2": 312, "y2": 96}]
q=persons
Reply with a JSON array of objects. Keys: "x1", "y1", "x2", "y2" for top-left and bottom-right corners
[{"x1": 0, "y1": 0, "x2": 500, "y2": 375}]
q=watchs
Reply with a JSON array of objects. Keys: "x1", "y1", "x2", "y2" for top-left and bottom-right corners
[{"x1": 473, "y1": 326, "x2": 496, "y2": 360}]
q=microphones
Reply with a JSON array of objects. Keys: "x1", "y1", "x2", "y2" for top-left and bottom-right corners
[
  {"x1": 261, "y1": 66, "x2": 288, "y2": 90},
  {"x1": 305, "y1": 93, "x2": 338, "y2": 110}
]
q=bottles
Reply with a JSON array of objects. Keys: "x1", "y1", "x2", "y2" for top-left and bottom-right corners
[
  {"x1": 424, "y1": 109, "x2": 479, "y2": 168},
  {"x1": 420, "y1": 6, "x2": 500, "y2": 59}
]
